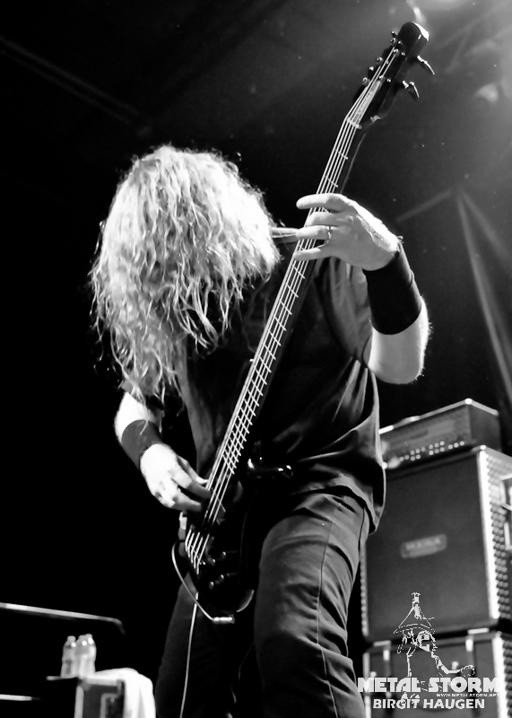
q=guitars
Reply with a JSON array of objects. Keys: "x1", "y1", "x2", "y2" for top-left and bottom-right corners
[{"x1": 171, "y1": 22, "x2": 434, "y2": 625}]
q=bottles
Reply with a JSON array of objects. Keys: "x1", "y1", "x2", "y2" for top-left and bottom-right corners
[{"x1": 61, "y1": 633, "x2": 97, "y2": 677}]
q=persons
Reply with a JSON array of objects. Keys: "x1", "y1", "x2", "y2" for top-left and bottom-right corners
[{"x1": 93, "y1": 143, "x2": 433, "y2": 718}]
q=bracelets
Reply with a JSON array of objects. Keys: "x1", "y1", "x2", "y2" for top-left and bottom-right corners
[
  {"x1": 122, "y1": 421, "x2": 167, "y2": 474},
  {"x1": 361, "y1": 239, "x2": 424, "y2": 334}
]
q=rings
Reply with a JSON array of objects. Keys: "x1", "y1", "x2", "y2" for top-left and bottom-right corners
[{"x1": 325, "y1": 223, "x2": 339, "y2": 240}]
